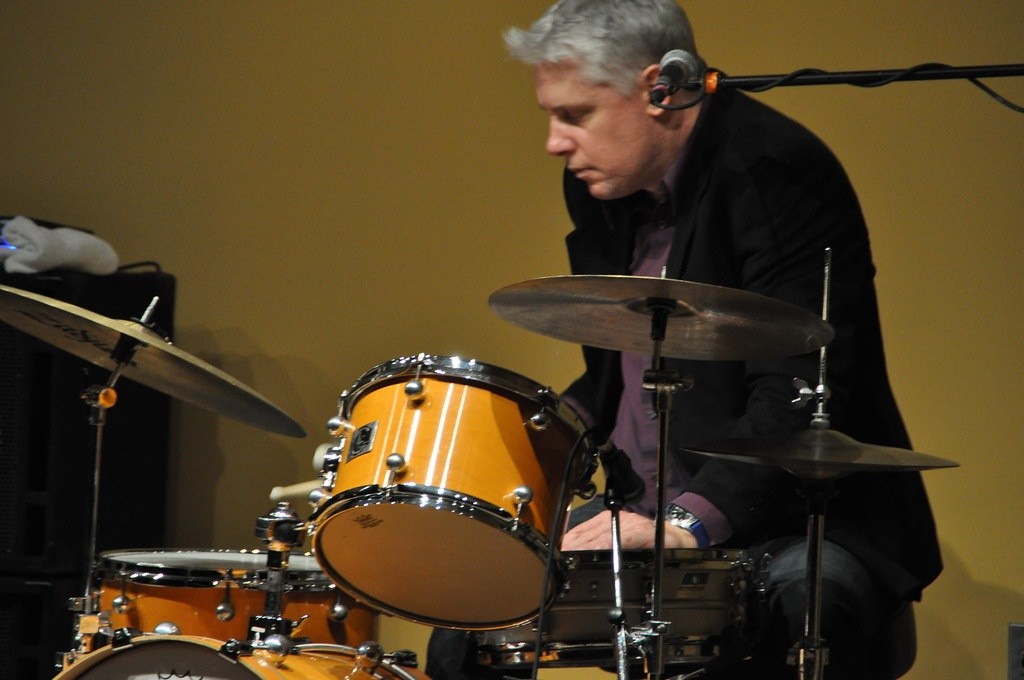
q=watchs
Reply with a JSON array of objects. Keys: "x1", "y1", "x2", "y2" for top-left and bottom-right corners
[{"x1": 666, "y1": 503, "x2": 709, "y2": 547}]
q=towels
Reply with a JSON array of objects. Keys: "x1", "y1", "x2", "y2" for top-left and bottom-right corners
[{"x1": 2, "y1": 216, "x2": 120, "y2": 276}]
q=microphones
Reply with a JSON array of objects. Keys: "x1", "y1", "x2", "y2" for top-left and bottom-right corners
[
  {"x1": 649, "y1": 48, "x2": 698, "y2": 105},
  {"x1": 589, "y1": 427, "x2": 647, "y2": 506}
]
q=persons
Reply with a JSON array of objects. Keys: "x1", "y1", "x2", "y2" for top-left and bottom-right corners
[{"x1": 504, "y1": 0, "x2": 943, "y2": 680}]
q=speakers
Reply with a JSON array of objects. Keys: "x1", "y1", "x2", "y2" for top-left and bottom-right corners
[
  {"x1": 0, "y1": 575, "x2": 82, "y2": 680},
  {"x1": 0, "y1": 271, "x2": 179, "y2": 582}
]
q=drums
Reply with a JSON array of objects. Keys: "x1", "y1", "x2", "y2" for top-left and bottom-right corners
[
  {"x1": 278, "y1": 552, "x2": 380, "y2": 650},
  {"x1": 51, "y1": 631, "x2": 433, "y2": 680},
  {"x1": 96, "y1": 546, "x2": 270, "y2": 642},
  {"x1": 472, "y1": 545, "x2": 749, "y2": 668},
  {"x1": 306, "y1": 352, "x2": 591, "y2": 631}
]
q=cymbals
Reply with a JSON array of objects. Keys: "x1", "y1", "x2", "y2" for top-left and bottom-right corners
[
  {"x1": 676, "y1": 429, "x2": 960, "y2": 481},
  {"x1": 487, "y1": 272, "x2": 836, "y2": 363},
  {"x1": 0, "y1": 283, "x2": 308, "y2": 439}
]
